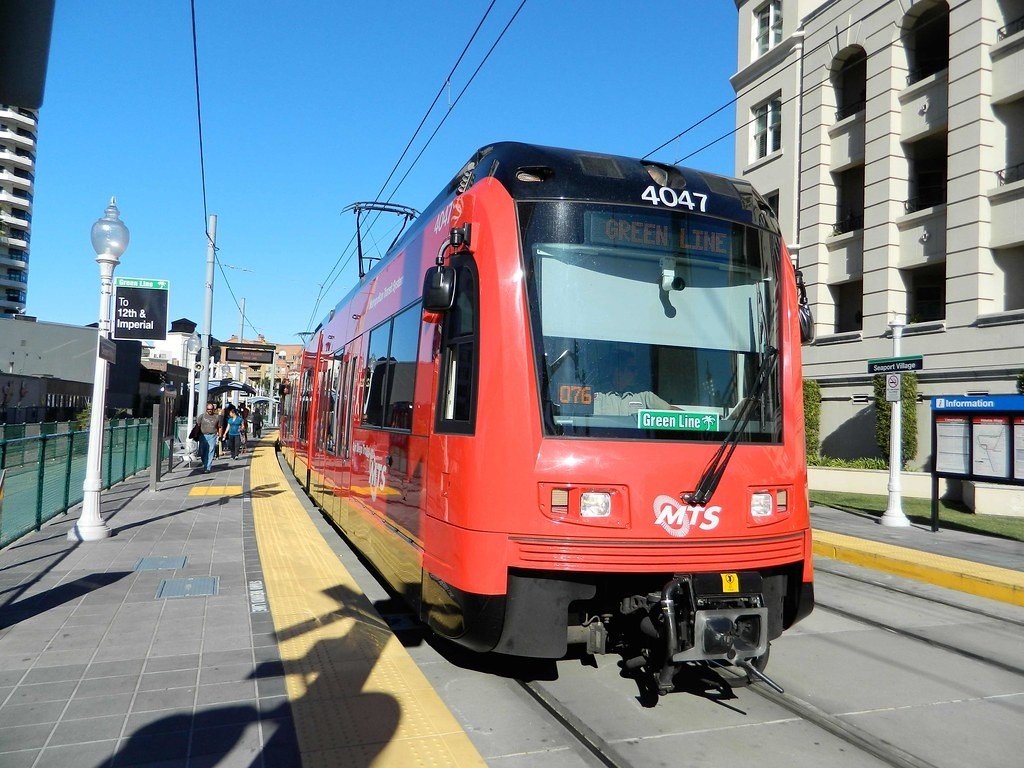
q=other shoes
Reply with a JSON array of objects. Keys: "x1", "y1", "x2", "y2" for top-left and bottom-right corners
[
  {"x1": 204, "y1": 470, "x2": 210, "y2": 473},
  {"x1": 235, "y1": 456, "x2": 238, "y2": 459}
]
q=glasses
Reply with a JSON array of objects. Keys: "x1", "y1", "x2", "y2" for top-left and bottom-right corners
[{"x1": 207, "y1": 409, "x2": 213, "y2": 410}]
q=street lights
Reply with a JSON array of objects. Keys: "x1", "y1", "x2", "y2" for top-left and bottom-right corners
[
  {"x1": 66, "y1": 193, "x2": 129, "y2": 541},
  {"x1": 182, "y1": 330, "x2": 203, "y2": 463}
]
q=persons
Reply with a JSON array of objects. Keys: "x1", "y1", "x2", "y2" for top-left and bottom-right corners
[
  {"x1": 215, "y1": 402, "x2": 263, "y2": 460},
  {"x1": 587, "y1": 351, "x2": 685, "y2": 416},
  {"x1": 198, "y1": 404, "x2": 222, "y2": 473}
]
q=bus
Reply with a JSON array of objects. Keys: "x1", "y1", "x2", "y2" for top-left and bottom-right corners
[{"x1": 279, "y1": 140, "x2": 817, "y2": 699}]
